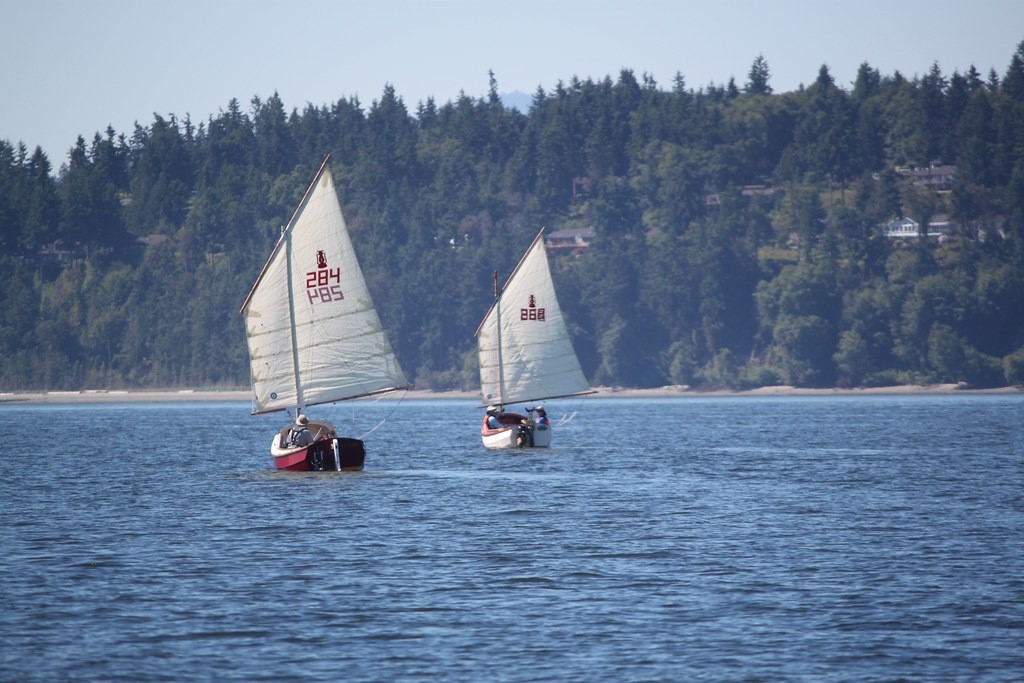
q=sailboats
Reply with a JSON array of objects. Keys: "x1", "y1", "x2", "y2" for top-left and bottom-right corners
[
  {"x1": 240, "y1": 153, "x2": 414, "y2": 472},
  {"x1": 472, "y1": 224, "x2": 600, "y2": 450}
]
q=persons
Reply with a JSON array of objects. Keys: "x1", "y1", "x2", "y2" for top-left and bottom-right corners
[
  {"x1": 484, "y1": 406, "x2": 507, "y2": 429},
  {"x1": 521, "y1": 406, "x2": 548, "y2": 430},
  {"x1": 286, "y1": 415, "x2": 313, "y2": 448}
]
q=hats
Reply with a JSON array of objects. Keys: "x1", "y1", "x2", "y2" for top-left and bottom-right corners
[
  {"x1": 296, "y1": 415, "x2": 309, "y2": 426},
  {"x1": 487, "y1": 406, "x2": 497, "y2": 412},
  {"x1": 535, "y1": 406, "x2": 546, "y2": 415}
]
